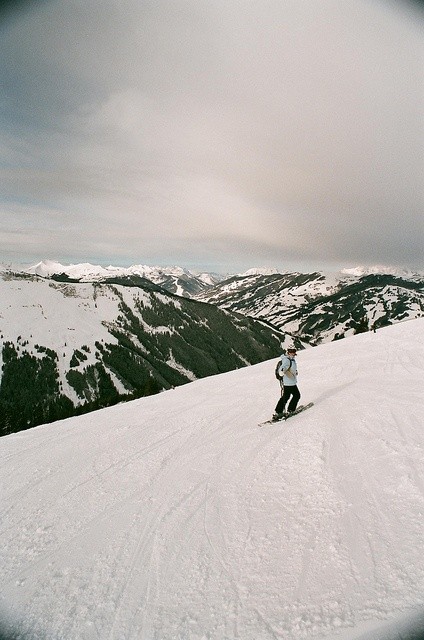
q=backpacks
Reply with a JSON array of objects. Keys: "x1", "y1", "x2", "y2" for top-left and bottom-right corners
[{"x1": 275, "y1": 358, "x2": 292, "y2": 380}]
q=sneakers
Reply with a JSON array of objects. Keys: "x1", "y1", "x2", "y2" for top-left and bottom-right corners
[
  {"x1": 277, "y1": 412, "x2": 283, "y2": 419},
  {"x1": 287, "y1": 409, "x2": 296, "y2": 415}
]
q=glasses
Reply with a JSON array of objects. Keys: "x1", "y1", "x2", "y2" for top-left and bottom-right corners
[{"x1": 288, "y1": 349, "x2": 296, "y2": 353}]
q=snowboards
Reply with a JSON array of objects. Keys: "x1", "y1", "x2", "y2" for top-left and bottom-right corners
[{"x1": 258, "y1": 401, "x2": 314, "y2": 429}]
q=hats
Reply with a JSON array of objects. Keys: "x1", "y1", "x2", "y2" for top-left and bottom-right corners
[{"x1": 287, "y1": 343, "x2": 297, "y2": 350}]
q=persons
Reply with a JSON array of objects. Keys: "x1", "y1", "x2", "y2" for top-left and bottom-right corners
[{"x1": 271, "y1": 346, "x2": 301, "y2": 421}]
